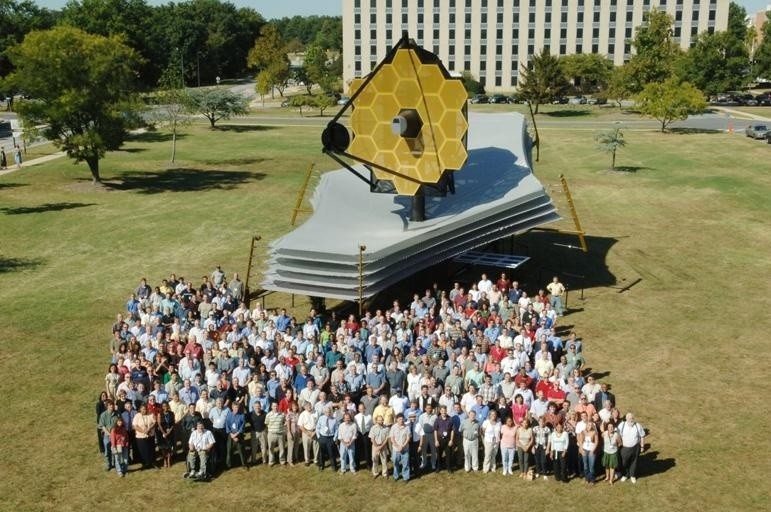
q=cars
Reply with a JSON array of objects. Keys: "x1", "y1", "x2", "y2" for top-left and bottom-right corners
[
  {"x1": 709, "y1": 90, "x2": 771, "y2": 107},
  {"x1": 746, "y1": 123, "x2": 770, "y2": 140},
  {"x1": 765, "y1": 130, "x2": 771, "y2": 144},
  {"x1": 466, "y1": 92, "x2": 610, "y2": 105},
  {"x1": 278, "y1": 91, "x2": 352, "y2": 108}
]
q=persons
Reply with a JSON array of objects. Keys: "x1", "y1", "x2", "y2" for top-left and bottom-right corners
[
  {"x1": 1, "y1": 147, "x2": 8, "y2": 170},
  {"x1": 95, "y1": 265, "x2": 646, "y2": 483},
  {"x1": 14, "y1": 144, "x2": 22, "y2": 168},
  {"x1": 216, "y1": 76, "x2": 220, "y2": 85}
]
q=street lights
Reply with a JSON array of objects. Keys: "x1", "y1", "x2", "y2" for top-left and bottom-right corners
[
  {"x1": 6, "y1": 97, "x2": 12, "y2": 112},
  {"x1": 747, "y1": 60, "x2": 757, "y2": 90},
  {"x1": 175, "y1": 46, "x2": 186, "y2": 91},
  {"x1": 216, "y1": 75, "x2": 220, "y2": 90}
]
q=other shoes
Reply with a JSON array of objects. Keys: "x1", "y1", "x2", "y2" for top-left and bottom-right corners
[
  {"x1": 320, "y1": 466, "x2": 357, "y2": 475},
  {"x1": 109, "y1": 460, "x2": 171, "y2": 478},
  {"x1": 226, "y1": 459, "x2": 318, "y2": 471},
  {"x1": 372, "y1": 475, "x2": 409, "y2": 483},
  {"x1": 519, "y1": 468, "x2": 637, "y2": 485},
  {"x1": 416, "y1": 466, "x2": 513, "y2": 477},
  {"x1": 188, "y1": 469, "x2": 196, "y2": 477},
  {"x1": 197, "y1": 472, "x2": 206, "y2": 480}
]
